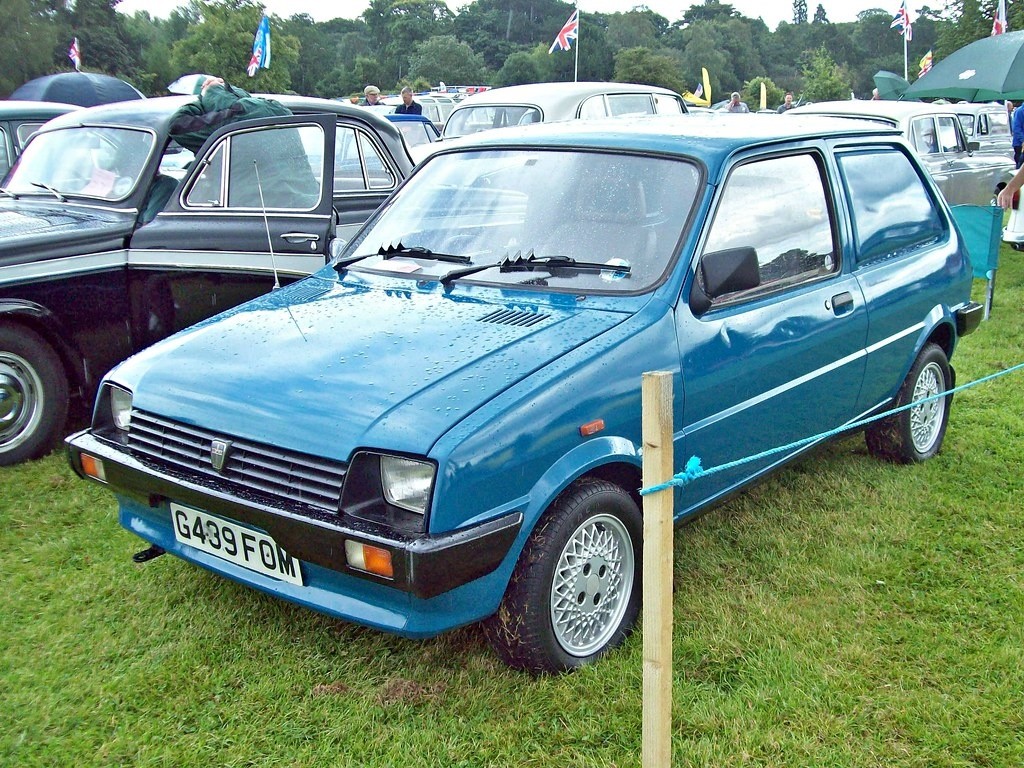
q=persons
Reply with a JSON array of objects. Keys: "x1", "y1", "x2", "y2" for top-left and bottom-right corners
[
  {"x1": 777, "y1": 93, "x2": 795, "y2": 114},
  {"x1": 360, "y1": 85, "x2": 386, "y2": 106},
  {"x1": 395, "y1": 86, "x2": 422, "y2": 115},
  {"x1": 724, "y1": 92, "x2": 749, "y2": 113},
  {"x1": 105, "y1": 140, "x2": 178, "y2": 229},
  {"x1": 872, "y1": 88, "x2": 879, "y2": 100},
  {"x1": 169, "y1": 78, "x2": 340, "y2": 261},
  {"x1": 997, "y1": 101, "x2": 1024, "y2": 209}
]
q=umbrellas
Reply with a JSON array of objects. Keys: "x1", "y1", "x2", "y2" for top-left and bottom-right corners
[
  {"x1": 167, "y1": 74, "x2": 216, "y2": 95},
  {"x1": 898, "y1": 30, "x2": 1024, "y2": 102},
  {"x1": 872, "y1": 70, "x2": 919, "y2": 102}
]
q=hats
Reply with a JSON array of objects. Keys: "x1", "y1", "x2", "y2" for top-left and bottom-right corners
[{"x1": 365, "y1": 86, "x2": 380, "y2": 96}]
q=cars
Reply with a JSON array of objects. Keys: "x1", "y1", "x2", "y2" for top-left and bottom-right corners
[
  {"x1": 65, "y1": 117, "x2": 986, "y2": 685},
  {"x1": 178, "y1": 68, "x2": 1020, "y2": 234},
  {"x1": 0, "y1": 96, "x2": 537, "y2": 465},
  {"x1": 0, "y1": 102, "x2": 206, "y2": 193}
]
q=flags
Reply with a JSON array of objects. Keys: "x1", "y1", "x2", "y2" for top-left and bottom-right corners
[
  {"x1": 549, "y1": 8, "x2": 578, "y2": 54},
  {"x1": 918, "y1": 49, "x2": 932, "y2": 78},
  {"x1": 991, "y1": 0, "x2": 1008, "y2": 36},
  {"x1": 760, "y1": 82, "x2": 766, "y2": 109},
  {"x1": 890, "y1": 0, "x2": 912, "y2": 40},
  {"x1": 67, "y1": 36, "x2": 81, "y2": 71}
]
